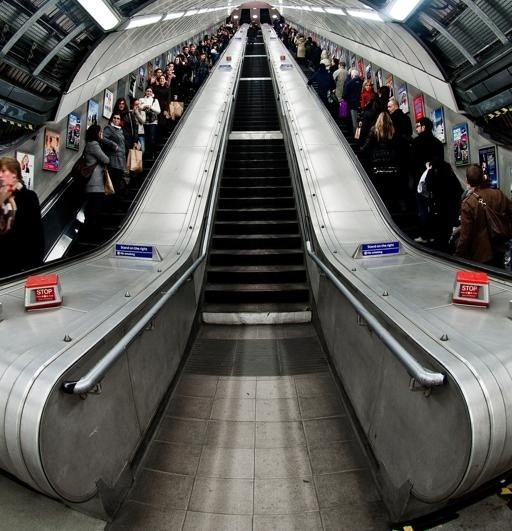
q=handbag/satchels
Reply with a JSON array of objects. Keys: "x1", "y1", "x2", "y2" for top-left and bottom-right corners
[
  {"x1": 126, "y1": 146, "x2": 145, "y2": 174},
  {"x1": 353, "y1": 125, "x2": 361, "y2": 139},
  {"x1": 169, "y1": 99, "x2": 184, "y2": 119},
  {"x1": 102, "y1": 164, "x2": 116, "y2": 196},
  {"x1": 147, "y1": 107, "x2": 159, "y2": 123},
  {"x1": 77, "y1": 158, "x2": 94, "y2": 182},
  {"x1": 482, "y1": 205, "x2": 512, "y2": 242},
  {"x1": 338, "y1": 98, "x2": 347, "y2": 118}
]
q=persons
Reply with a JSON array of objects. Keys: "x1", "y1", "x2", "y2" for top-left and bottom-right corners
[
  {"x1": 247, "y1": 19, "x2": 259, "y2": 45},
  {"x1": 22, "y1": 154, "x2": 31, "y2": 172},
  {"x1": 274, "y1": 19, "x2": 512, "y2": 272},
  {"x1": 83, "y1": 20, "x2": 237, "y2": 265},
  {"x1": 0, "y1": 156, "x2": 44, "y2": 279}
]
